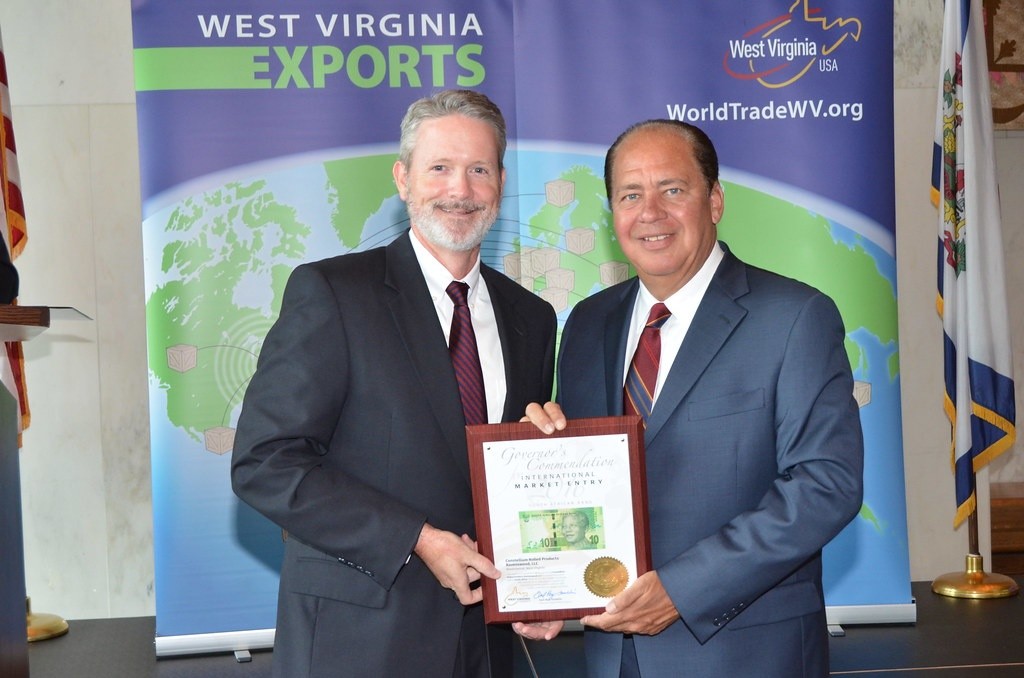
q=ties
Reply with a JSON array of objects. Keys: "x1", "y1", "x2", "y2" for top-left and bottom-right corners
[
  {"x1": 445, "y1": 281, "x2": 488, "y2": 426},
  {"x1": 623, "y1": 302, "x2": 673, "y2": 432}
]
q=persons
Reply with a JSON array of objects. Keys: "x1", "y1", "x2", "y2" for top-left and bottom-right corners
[
  {"x1": 230, "y1": 88, "x2": 559, "y2": 678},
  {"x1": 517, "y1": 117, "x2": 864, "y2": 677}
]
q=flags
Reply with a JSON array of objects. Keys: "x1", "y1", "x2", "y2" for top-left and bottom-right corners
[{"x1": 934, "y1": 1, "x2": 1018, "y2": 532}]
